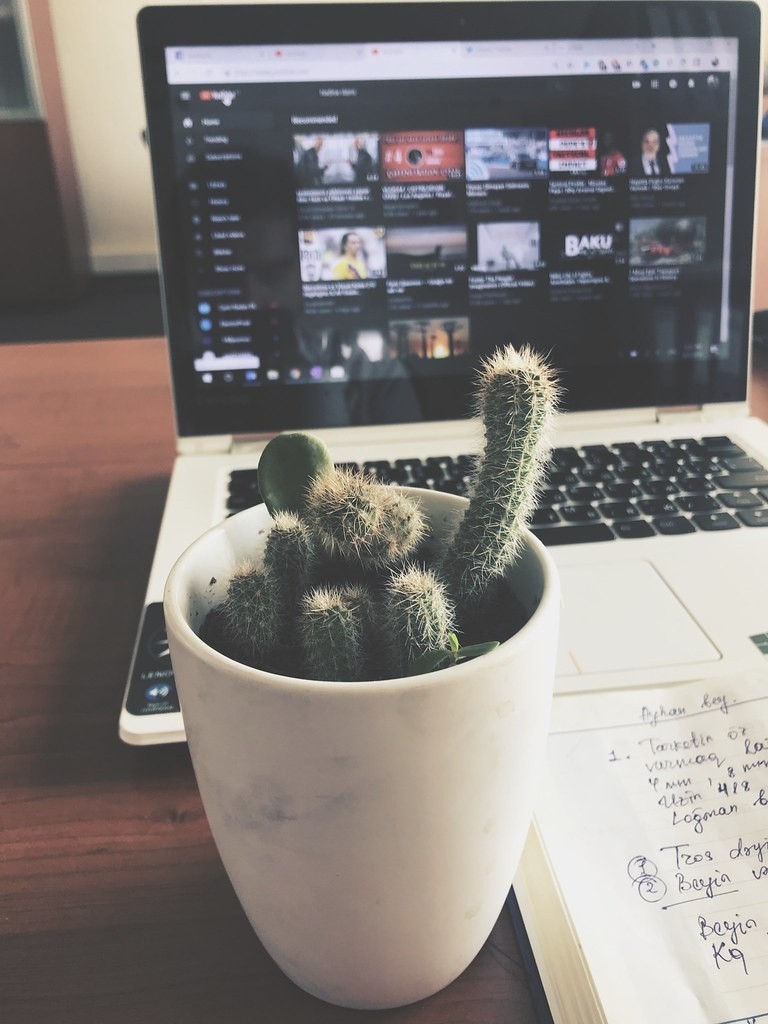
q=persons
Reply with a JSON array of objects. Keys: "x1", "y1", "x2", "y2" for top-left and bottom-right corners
[
  {"x1": 348, "y1": 138, "x2": 373, "y2": 182},
  {"x1": 297, "y1": 137, "x2": 329, "y2": 186},
  {"x1": 300, "y1": 230, "x2": 368, "y2": 280},
  {"x1": 629, "y1": 129, "x2": 671, "y2": 176}
]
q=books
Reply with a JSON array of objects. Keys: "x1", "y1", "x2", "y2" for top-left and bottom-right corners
[{"x1": 504, "y1": 668, "x2": 768, "y2": 1024}]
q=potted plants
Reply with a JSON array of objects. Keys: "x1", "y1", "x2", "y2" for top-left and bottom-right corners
[{"x1": 162, "y1": 340, "x2": 562, "y2": 1013}]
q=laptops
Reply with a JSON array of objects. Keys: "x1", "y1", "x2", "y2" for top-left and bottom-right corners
[{"x1": 119, "y1": 0, "x2": 768, "y2": 746}]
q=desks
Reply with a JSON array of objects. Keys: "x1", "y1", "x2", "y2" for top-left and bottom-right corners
[{"x1": 0, "y1": 337, "x2": 768, "y2": 1024}]
